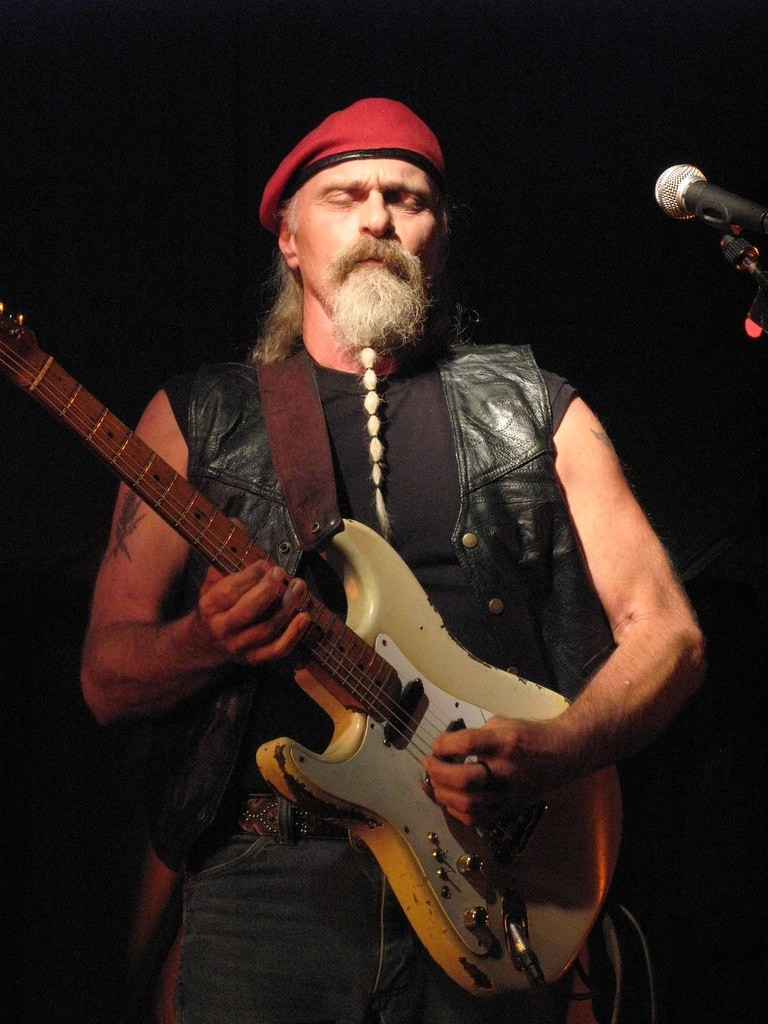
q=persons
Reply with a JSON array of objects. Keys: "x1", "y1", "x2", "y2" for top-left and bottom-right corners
[{"x1": 77, "y1": 96, "x2": 704, "y2": 1024}]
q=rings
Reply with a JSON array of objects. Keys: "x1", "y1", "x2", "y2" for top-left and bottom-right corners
[{"x1": 478, "y1": 760, "x2": 493, "y2": 788}]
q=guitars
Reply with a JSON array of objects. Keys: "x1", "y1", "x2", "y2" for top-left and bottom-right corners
[{"x1": 1, "y1": 298, "x2": 624, "y2": 997}]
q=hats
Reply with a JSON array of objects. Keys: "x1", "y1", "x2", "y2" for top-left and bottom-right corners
[{"x1": 259, "y1": 96, "x2": 446, "y2": 235}]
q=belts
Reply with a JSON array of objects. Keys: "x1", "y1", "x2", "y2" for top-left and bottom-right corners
[{"x1": 229, "y1": 792, "x2": 372, "y2": 852}]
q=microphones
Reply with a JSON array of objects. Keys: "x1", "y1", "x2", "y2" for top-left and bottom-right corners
[{"x1": 654, "y1": 164, "x2": 768, "y2": 238}]
